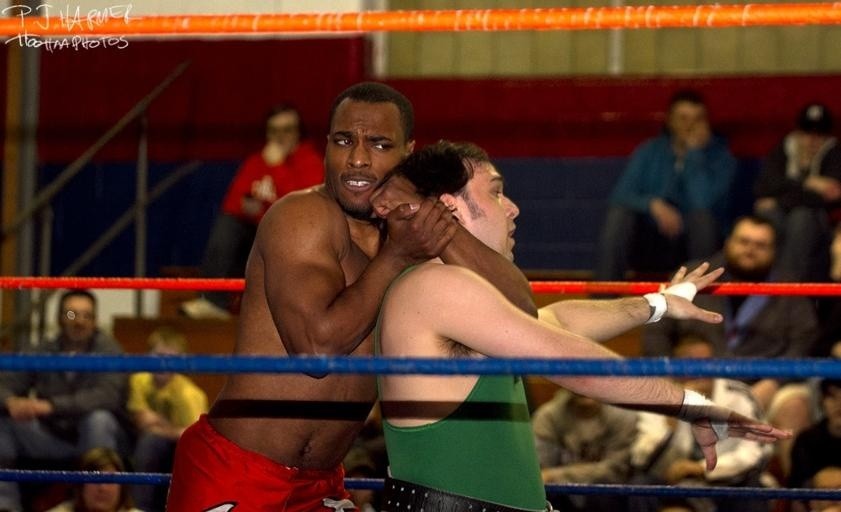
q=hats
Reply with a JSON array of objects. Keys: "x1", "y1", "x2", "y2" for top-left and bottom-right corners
[{"x1": 796, "y1": 103, "x2": 834, "y2": 136}]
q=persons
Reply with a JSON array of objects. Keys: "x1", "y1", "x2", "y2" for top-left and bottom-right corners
[
  {"x1": 165, "y1": 81, "x2": 417, "y2": 512},
  {"x1": 531, "y1": 88, "x2": 841, "y2": 511},
  {"x1": 178, "y1": 101, "x2": 325, "y2": 321},
  {"x1": 375, "y1": 139, "x2": 793, "y2": 512}
]
